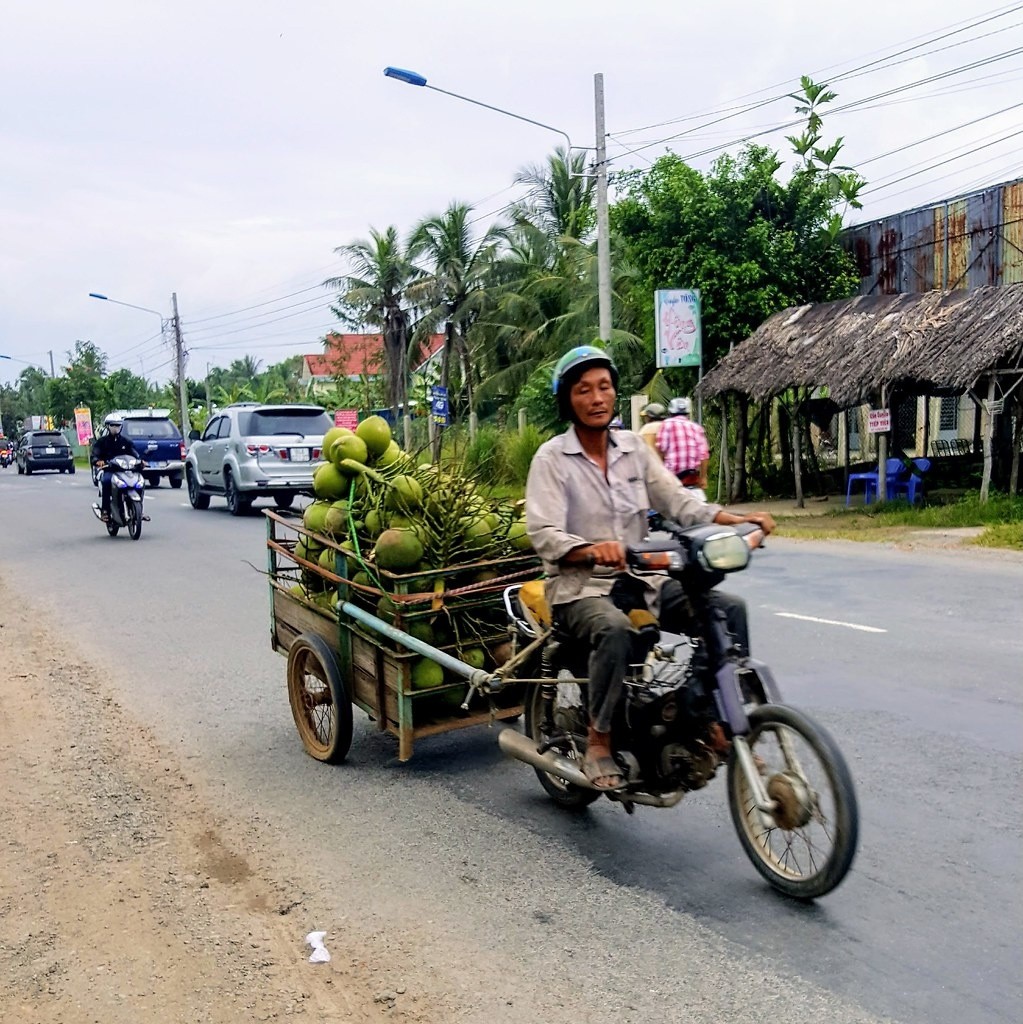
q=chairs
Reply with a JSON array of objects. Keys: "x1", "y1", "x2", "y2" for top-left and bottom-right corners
[
  {"x1": 931, "y1": 439, "x2": 951, "y2": 458},
  {"x1": 950, "y1": 438, "x2": 971, "y2": 455},
  {"x1": 846, "y1": 459, "x2": 906, "y2": 506},
  {"x1": 886, "y1": 459, "x2": 930, "y2": 504}
]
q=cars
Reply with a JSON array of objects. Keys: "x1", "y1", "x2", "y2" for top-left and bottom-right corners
[
  {"x1": 16, "y1": 429, "x2": 77, "y2": 475},
  {"x1": 91, "y1": 408, "x2": 186, "y2": 489}
]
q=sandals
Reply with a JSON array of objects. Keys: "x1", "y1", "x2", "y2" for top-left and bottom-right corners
[{"x1": 578, "y1": 755, "x2": 629, "y2": 792}]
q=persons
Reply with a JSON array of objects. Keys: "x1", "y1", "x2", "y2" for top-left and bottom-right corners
[
  {"x1": 656, "y1": 397, "x2": 709, "y2": 490},
  {"x1": 90, "y1": 414, "x2": 150, "y2": 522},
  {"x1": 0, "y1": 433, "x2": 8, "y2": 451},
  {"x1": 639, "y1": 403, "x2": 665, "y2": 461},
  {"x1": 526, "y1": 346, "x2": 775, "y2": 790}
]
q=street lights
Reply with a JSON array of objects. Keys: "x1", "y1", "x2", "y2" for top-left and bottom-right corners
[
  {"x1": 0, "y1": 350, "x2": 54, "y2": 379},
  {"x1": 89, "y1": 292, "x2": 192, "y2": 452},
  {"x1": 384, "y1": 67, "x2": 609, "y2": 342}
]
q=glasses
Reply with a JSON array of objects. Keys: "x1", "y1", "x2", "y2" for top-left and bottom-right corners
[{"x1": 109, "y1": 424, "x2": 121, "y2": 426}]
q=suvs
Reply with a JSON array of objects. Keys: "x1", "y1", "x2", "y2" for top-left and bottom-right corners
[{"x1": 186, "y1": 401, "x2": 336, "y2": 515}]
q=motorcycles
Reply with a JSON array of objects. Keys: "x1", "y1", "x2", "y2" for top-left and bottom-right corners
[
  {"x1": 91, "y1": 455, "x2": 152, "y2": 540},
  {"x1": 498, "y1": 522, "x2": 861, "y2": 902},
  {"x1": 0, "y1": 450, "x2": 10, "y2": 469}
]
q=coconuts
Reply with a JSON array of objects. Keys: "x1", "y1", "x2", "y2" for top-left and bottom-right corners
[{"x1": 289, "y1": 415, "x2": 535, "y2": 691}]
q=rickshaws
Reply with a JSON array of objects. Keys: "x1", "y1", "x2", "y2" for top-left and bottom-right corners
[{"x1": 261, "y1": 507, "x2": 544, "y2": 764}]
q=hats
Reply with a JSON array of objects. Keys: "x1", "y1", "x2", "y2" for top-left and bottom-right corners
[
  {"x1": 640, "y1": 403, "x2": 666, "y2": 419},
  {"x1": 668, "y1": 398, "x2": 691, "y2": 414}
]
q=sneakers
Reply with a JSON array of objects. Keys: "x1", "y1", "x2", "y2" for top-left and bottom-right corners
[{"x1": 101, "y1": 510, "x2": 109, "y2": 522}]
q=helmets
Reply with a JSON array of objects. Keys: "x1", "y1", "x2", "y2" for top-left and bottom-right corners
[
  {"x1": 104, "y1": 413, "x2": 124, "y2": 425},
  {"x1": 553, "y1": 345, "x2": 619, "y2": 395}
]
